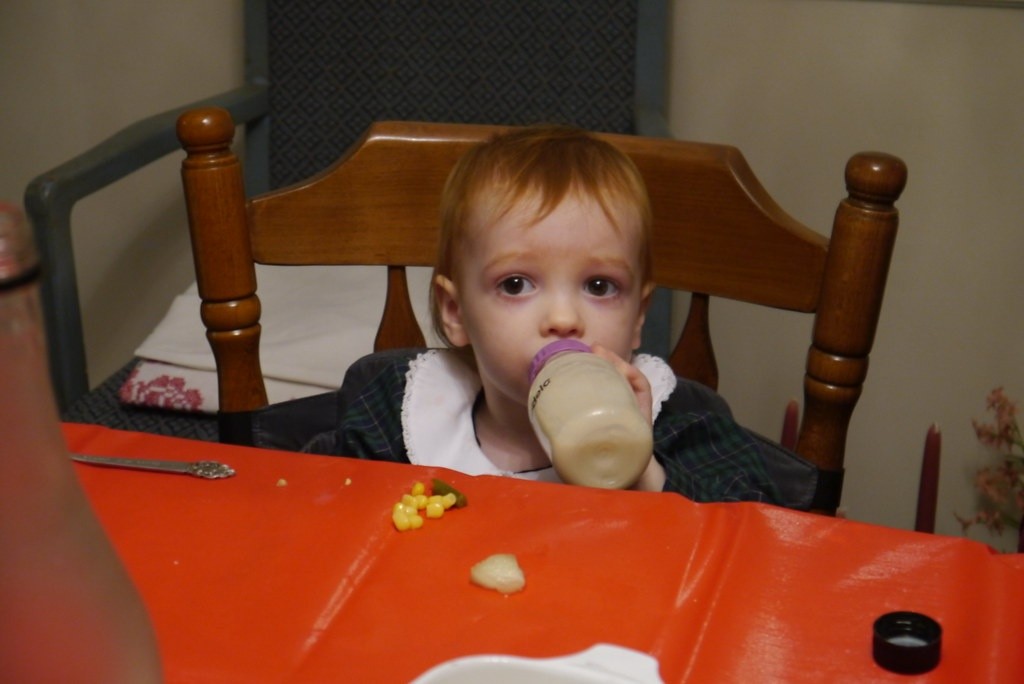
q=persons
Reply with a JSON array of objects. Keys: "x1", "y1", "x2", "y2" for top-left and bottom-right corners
[
  {"x1": 0, "y1": 205, "x2": 164, "y2": 684},
  {"x1": 293, "y1": 127, "x2": 775, "y2": 507}
]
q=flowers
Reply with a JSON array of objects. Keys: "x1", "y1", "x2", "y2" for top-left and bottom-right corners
[{"x1": 952, "y1": 385, "x2": 1024, "y2": 552}]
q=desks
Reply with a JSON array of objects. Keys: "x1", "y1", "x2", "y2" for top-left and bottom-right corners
[{"x1": 57, "y1": 423, "x2": 1024, "y2": 684}]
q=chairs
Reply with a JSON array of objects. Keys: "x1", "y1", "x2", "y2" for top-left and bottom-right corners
[
  {"x1": 176, "y1": 108, "x2": 906, "y2": 515},
  {"x1": 25, "y1": 0, "x2": 674, "y2": 454}
]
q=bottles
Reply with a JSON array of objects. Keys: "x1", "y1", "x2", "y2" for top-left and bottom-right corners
[{"x1": 524, "y1": 337, "x2": 655, "y2": 492}]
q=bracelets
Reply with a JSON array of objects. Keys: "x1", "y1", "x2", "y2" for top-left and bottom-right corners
[{"x1": 0, "y1": 264, "x2": 41, "y2": 293}]
